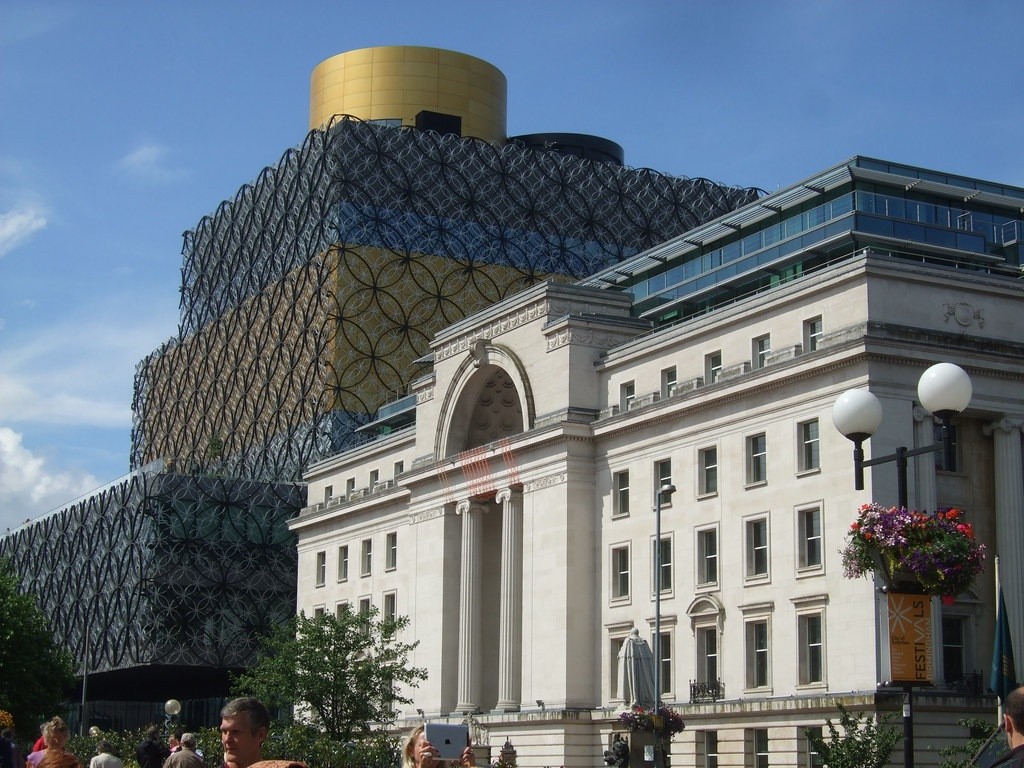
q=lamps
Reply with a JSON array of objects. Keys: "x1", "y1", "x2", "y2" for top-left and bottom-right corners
[
  {"x1": 88, "y1": 726, "x2": 101, "y2": 736},
  {"x1": 163, "y1": 699, "x2": 183, "y2": 732}
]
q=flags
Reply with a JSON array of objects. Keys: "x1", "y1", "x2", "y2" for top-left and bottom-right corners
[{"x1": 989, "y1": 580, "x2": 1019, "y2": 719}]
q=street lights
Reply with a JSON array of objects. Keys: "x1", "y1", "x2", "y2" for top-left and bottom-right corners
[
  {"x1": 829, "y1": 357, "x2": 973, "y2": 768},
  {"x1": 652, "y1": 483, "x2": 678, "y2": 718}
]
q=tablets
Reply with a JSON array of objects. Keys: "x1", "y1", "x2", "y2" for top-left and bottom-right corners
[{"x1": 423, "y1": 723, "x2": 469, "y2": 761}]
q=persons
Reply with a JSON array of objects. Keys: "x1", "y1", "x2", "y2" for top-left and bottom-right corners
[
  {"x1": 988, "y1": 686, "x2": 1024, "y2": 768},
  {"x1": 137, "y1": 725, "x2": 206, "y2": 768},
  {"x1": 618, "y1": 627, "x2": 664, "y2": 710},
  {"x1": 400, "y1": 722, "x2": 477, "y2": 768},
  {"x1": 89, "y1": 740, "x2": 123, "y2": 768},
  {"x1": 0, "y1": 711, "x2": 82, "y2": 768},
  {"x1": 220, "y1": 697, "x2": 269, "y2": 768}
]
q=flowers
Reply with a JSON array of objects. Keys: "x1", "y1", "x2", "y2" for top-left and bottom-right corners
[{"x1": 841, "y1": 500, "x2": 987, "y2": 605}]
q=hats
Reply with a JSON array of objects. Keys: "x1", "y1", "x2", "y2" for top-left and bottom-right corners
[{"x1": 180, "y1": 733, "x2": 195, "y2": 745}]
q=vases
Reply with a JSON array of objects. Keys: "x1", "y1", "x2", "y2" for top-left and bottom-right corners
[{"x1": 879, "y1": 554, "x2": 920, "y2": 585}]
